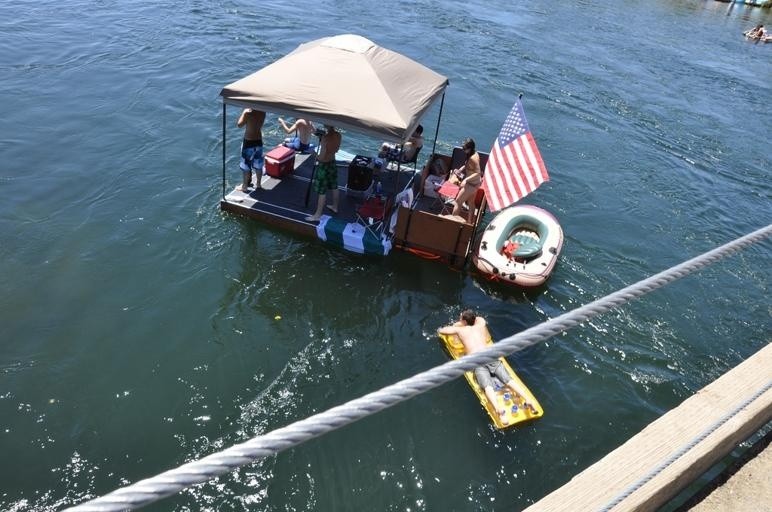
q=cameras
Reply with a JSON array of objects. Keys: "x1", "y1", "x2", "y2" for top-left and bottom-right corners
[{"x1": 314, "y1": 128, "x2": 326, "y2": 138}]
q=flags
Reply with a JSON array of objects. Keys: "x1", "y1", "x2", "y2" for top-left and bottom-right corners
[{"x1": 482, "y1": 99, "x2": 550, "y2": 213}]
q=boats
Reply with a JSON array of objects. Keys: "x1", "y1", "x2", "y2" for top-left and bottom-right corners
[
  {"x1": 742, "y1": 30, "x2": 772, "y2": 45},
  {"x1": 473, "y1": 200, "x2": 565, "y2": 290},
  {"x1": 209, "y1": 140, "x2": 492, "y2": 267}
]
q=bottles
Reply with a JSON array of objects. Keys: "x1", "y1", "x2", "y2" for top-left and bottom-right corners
[
  {"x1": 449, "y1": 168, "x2": 464, "y2": 180},
  {"x1": 376, "y1": 182, "x2": 382, "y2": 193}
]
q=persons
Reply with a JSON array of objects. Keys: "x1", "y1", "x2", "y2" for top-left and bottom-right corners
[
  {"x1": 451, "y1": 137, "x2": 482, "y2": 223},
  {"x1": 304, "y1": 125, "x2": 342, "y2": 222},
  {"x1": 750, "y1": 24, "x2": 764, "y2": 40},
  {"x1": 439, "y1": 309, "x2": 539, "y2": 425},
  {"x1": 237, "y1": 109, "x2": 267, "y2": 191},
  {"x1": 378, "y1": 125, "x2": 423, "y2": 163},
  {"x1": 277, "y1": 117, "x2": 316, "y2": 150}
]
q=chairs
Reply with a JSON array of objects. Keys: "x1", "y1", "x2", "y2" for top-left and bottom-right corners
[
  {"x1": 389, "y1": 145, "x2": 424, "y2": 193},
  {"x1": 354, "y1": 191, "x2": 394, "y2": 242}
]
